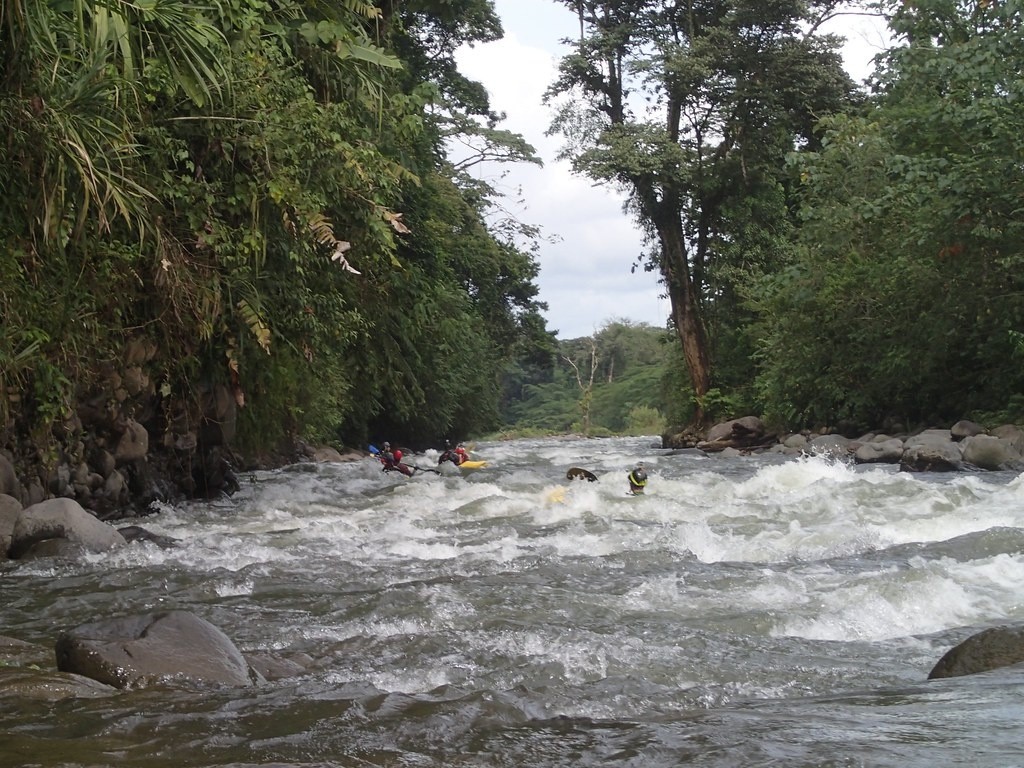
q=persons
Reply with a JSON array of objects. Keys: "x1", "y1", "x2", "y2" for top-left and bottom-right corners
[
  {"x1": 625, "y1": 467, "x2": 651, "y2": 498},
  {"x1": 373, "y1": 441, "x2": 470, "y2": 467},
  {"x1": 381, "y1": 450, "x2": 419, "y2": 476}
]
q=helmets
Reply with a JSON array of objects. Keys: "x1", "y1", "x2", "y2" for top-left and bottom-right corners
[
  {"x1": 628, "y1": 468, "x2": 648, "y2": 486},
  {"x1": 455, "y1": 448, "x2": 464, "y2": 453},
  {"x1": 393, "y1": 450, "x2": 402, "y2": 459}
]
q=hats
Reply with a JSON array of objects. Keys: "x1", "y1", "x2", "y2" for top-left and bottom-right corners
[
  {"x1": 445, "y1": 442, "x2": 451, "y2": 450},
  {"x1": 383, "y1": 441, "x2": 390, "y2": 447}
]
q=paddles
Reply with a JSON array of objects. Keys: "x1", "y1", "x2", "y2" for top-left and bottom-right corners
[
  {"x1": 464, "y1": 442, "x2": 476, "y2": 455},
  {"x1": 567, "y1": 468, "x2": 602, "y2": 485},
  {"x1": 367, "y1": 445, "x2": 382, "y2": 454},
  {"x1": 367, "y1": 453, "x2": 442, "y2": 477}
]
